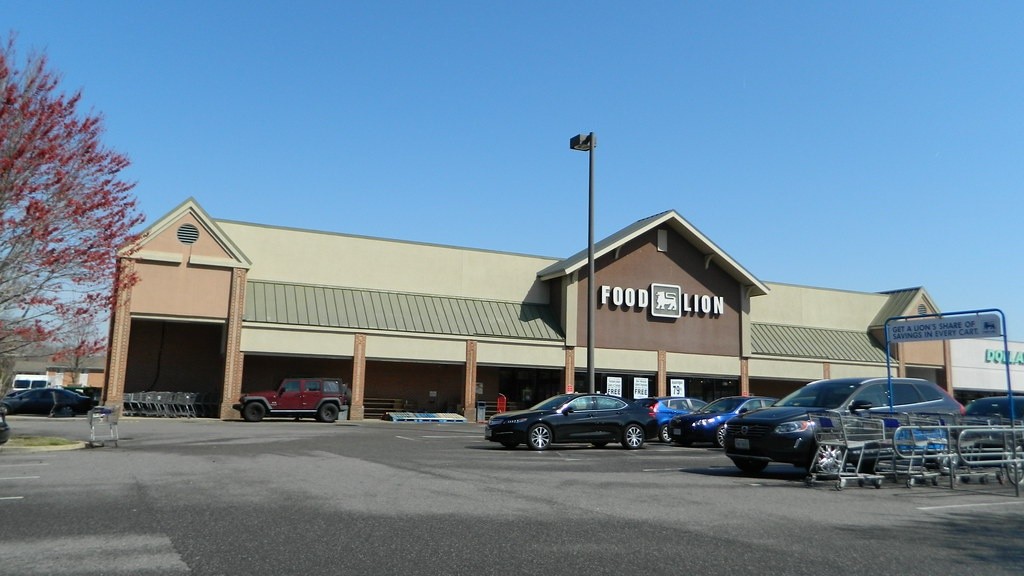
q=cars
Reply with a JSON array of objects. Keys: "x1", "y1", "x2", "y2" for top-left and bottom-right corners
[
  {"x1": 0, "y1": 387, "x2": 95, "y2": 418},
  {"x1": 484, "y1": 392, "x2": 661, "y2": 450},
  {"x1": 667, "y1": 396, "x2": 782, "y2": 448},
  {"x1": 0, "y1": 410, "x2": 10, "y2": 446},
  {"x1": 961, "y1": 396, "x2": 1024, "y2": 469}
]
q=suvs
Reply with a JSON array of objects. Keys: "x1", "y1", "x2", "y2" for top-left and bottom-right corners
[
  {"x1": 633, "y1": 396, "x2": 719, "y2": 444},
  {"x1": 232, "y1": 377, "x2": 352, "y2": 423},
  {"x1": 722, "y1": 376, "x2": 968, "y2": 482}
]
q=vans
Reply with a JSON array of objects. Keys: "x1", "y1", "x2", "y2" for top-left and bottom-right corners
[{"x1": 12, "y1": 374, "x2": 51, "y2": 393}]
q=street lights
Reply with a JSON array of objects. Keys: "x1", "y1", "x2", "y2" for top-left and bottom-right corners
[{"x1": 569, "y1": 131, "x2": 598, "y2": 396}]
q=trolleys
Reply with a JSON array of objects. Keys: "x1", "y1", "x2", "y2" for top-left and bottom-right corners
[
  {"x1": 869, "y1": 409, "x2": 1024, "y2": 489},
  {"x1": 122, "y1": 391, "x2": 199, "y2": 419},
  {"x1": 804, "y1": 411, "x2": 886, "y2": 491},
  {"x1": 87, "y1": 404, "x2": 121, "y2": 448}
]
